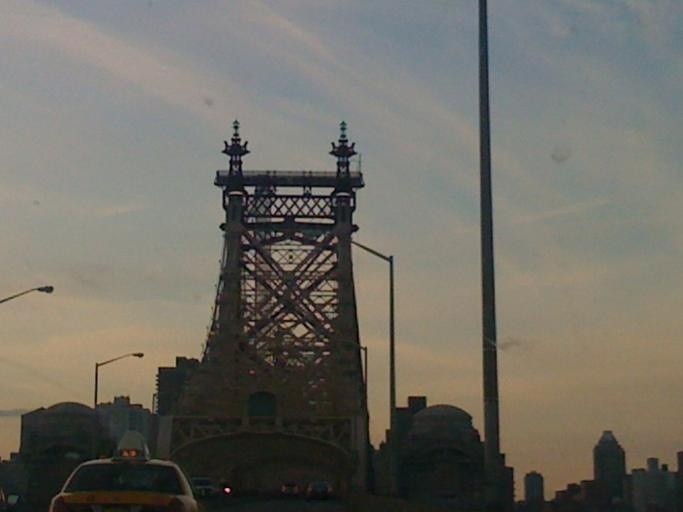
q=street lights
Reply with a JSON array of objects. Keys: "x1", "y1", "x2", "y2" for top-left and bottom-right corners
[
  {"x1": 264, "y1": 223, "x2": 396, "y2": 421},
  {"x1": 92, "y1": 350, "x2": 144, "y2": 404},
  {"x1": 0, "y1": 285, "x2": 54, "y2": 306}
]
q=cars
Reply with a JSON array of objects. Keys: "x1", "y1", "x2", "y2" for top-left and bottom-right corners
[
  {"x1": 52, "y1": 456, "x2": 198, "y2": 510},
  {"x1": 191, "y1": 473, "x2": 334, "y2": 500}
]
